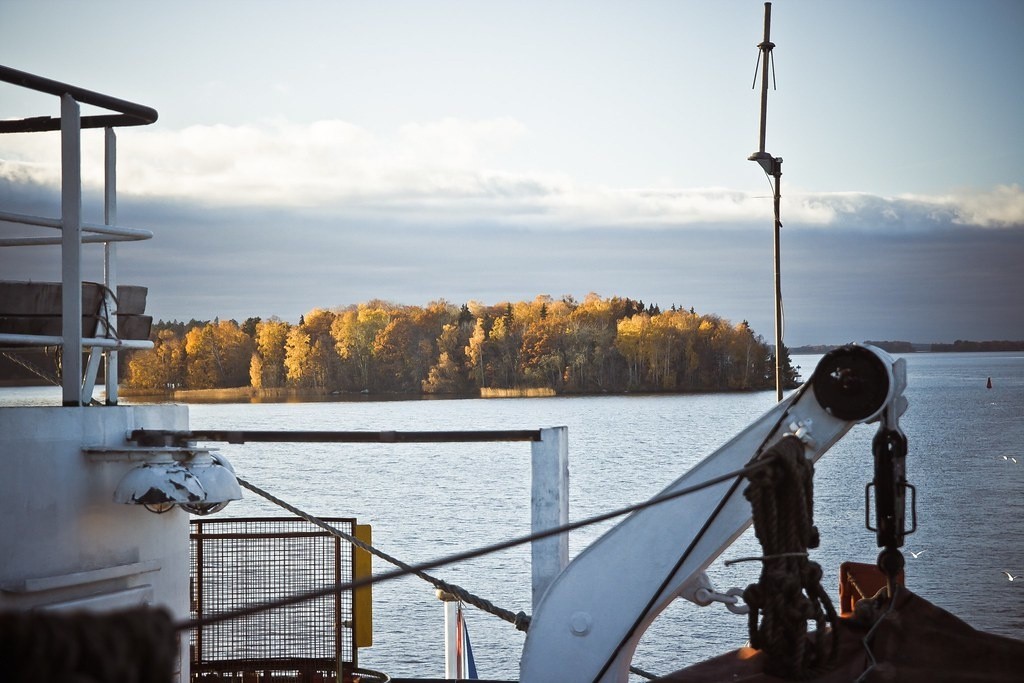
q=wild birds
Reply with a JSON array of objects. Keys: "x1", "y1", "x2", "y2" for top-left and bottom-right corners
[
  {"x1": 996, "y1": 454, "x2": 1017, "y2": 465},
  {"x1": 909, "y1": 549, "x2": 929, "y2": 559},
  {"x1": 1001, "y1": 571, "x2": 1023, "y2": 583}
]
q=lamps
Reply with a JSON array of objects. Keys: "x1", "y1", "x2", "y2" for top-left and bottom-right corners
[
  {"x1": 178, "y1": 451, "x2": 244, "y2": 516},
  {"x1": 89, "y1": 451, "x2": 207, "y2": 513}
]
